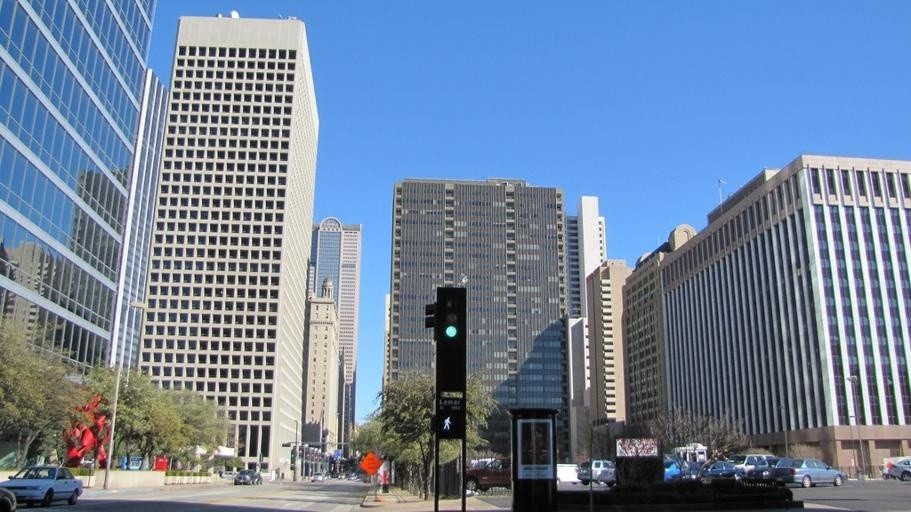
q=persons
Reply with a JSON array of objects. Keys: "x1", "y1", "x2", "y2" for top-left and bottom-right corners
[{"x1": 379, "y1": 468, "x2": 390, "y2": 493}]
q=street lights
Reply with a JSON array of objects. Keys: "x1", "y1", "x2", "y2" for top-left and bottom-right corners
[
  {"x1": 292, "y1": 419, "x2": 298, "y2": 480},
  {"x1": 846, "y1": 375, "x2": 864, "y2": 480},
  {"x1": 101, "y1": 301, "x2": 150, "y2": 490}
]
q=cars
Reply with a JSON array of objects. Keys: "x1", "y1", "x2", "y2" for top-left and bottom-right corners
[
  {"x1": 465, "y1": 456, "x2": 512, "y2": 491},
  {"x1": 233, "y1": 469, "x2": 264, "y2": 486},
  {"x1": 660, "y1": 455, "x2": 843, "y2": 489},
  {"x1": 0, "y1": 486, "x2": 18, "y2": 512},
  {"x1": 601, "y1": 462, "x2": 616, "y2": 487},
  {"x1": 1, "y1": 463, "x2": 84, "y2": 507},
  {"x1": 879, "y1": 459, "x2": 910, "y2": 482},
  {"x1": 311, "y1": 471, "x2": 356, "y2": 483},
  {"x1": 221, "y1": 471, "x2": 238, "y2": 479}
]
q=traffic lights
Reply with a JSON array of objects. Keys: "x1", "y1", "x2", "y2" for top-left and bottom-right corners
[
  {"x1": 281, "y1": 443, "x2": 290, "y2": 448},
  {"x1": 444, "y1": 297, "x2": 459, "y2": 342},
  {"x1": 424, "y1": 302, "x2": 437, "y2": 343}
]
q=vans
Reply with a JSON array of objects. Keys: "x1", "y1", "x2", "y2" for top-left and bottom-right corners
[{"x1": 577, "y1": 459, "x2": 614, "y2": 485}]
